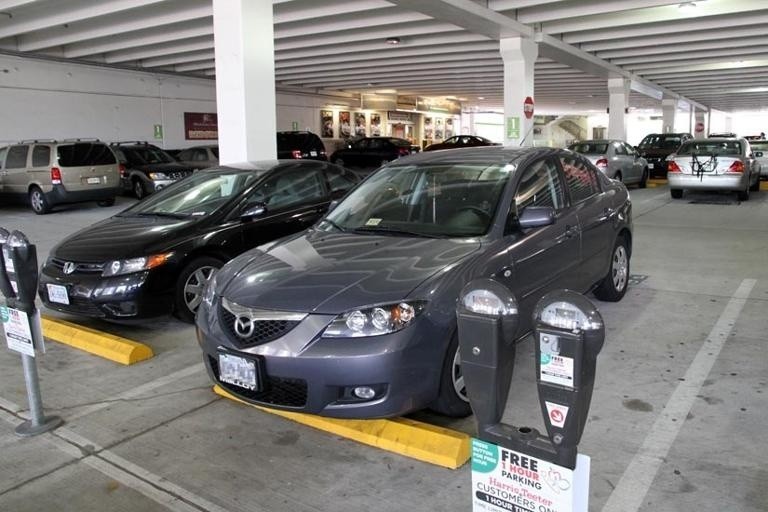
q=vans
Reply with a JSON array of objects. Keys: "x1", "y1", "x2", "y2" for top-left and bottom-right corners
[{"x1": 1, "y1": 138, "x2": 127, "y2": 215}]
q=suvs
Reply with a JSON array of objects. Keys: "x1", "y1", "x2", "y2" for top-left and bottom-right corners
[
  {"x1": 111, "y1": 143, "x2": 194, "y2": 199},
  {"x1": 277, "y1": 129, "x2": 328, "y2": 160}
]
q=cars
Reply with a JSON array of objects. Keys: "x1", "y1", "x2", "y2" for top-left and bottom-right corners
[
  {"x1": 635, "y1": 132, "x2": 693, "y2": 178},
  {"x1": 423, "y1": 134, "x2": 503, "y2": 151},
  {"x1": 175, "y1": 145, "x2": 219, "y2": 170},
  {"x1": 666, "y1": 135, "x2": 768, "y2": 200},
  {"x1": 194, "y1": 148, "x2": 633, "y2": 419},
  {"x1": 330, "y1": 137, "x2": 420, "y2": 168},
  {"x1": 39, "y1": 158, "x2": 368, "y2": 320},
  {"x1": 566, "y1": 140, "x2": 649, "y2": 189}
]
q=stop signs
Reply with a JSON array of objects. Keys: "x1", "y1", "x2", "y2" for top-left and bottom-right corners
[{"x1": 524, "y1": 97, "x2": 534, "y2": 119}]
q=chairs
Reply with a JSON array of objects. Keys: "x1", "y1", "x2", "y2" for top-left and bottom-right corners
[
  {"x1": 695, "y1": 143, "x2": 738, "y2": 154},
  {"x1": 418, "y1": 179, "x2": 472, "y2": 220},
  {"x1": 588, "y1": 145, "x2": 597, "y2": 153},
  {"x1": 240, "y1": 178, "x2": 305, "y2": 212}
]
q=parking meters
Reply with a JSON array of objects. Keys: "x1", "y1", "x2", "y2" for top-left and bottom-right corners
[
  {"x1": 0, "y1": 228, "x2": 43, "y2": 425},
  {"x1": 457, "y1": 280, "x2": 606, "y2": 512}
]
